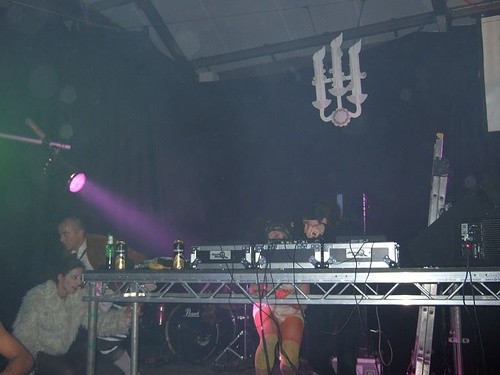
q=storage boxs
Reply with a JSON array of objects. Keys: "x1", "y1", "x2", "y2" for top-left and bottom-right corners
[{"x1": 191, "y1": 242, "x2": 400, "y2": 269}]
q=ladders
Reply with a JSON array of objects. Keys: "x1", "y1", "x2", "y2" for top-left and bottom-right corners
[{"x1": 408, "y1": 132, "x2": 451, "y2": 375}]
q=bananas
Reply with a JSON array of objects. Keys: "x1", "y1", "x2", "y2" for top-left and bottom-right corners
[{"x1": 148, "y1": 262, "x2": 164, "y2": 269}]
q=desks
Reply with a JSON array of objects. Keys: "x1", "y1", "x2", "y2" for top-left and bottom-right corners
[{"x1": 82, "y1": 271, "x2": 500, "y2": 375}]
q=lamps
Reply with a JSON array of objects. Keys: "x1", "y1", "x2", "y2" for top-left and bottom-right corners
[{"x1": 40, "y1": 147, "x2": 88, "y2": 192}]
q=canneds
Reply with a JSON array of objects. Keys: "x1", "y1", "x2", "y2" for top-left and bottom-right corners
[
  {"x1": 172, "y1": 239, "x2": 184, "y2": 270},
  {"x1": 115, "y1": 240, "x2": 126, "y2": 270}
]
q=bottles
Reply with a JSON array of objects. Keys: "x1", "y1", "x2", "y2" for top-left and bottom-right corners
[{"x1": 104, "y1": 233, "x2": 116, "y2": 271}]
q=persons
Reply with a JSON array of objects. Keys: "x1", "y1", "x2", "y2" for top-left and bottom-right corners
[
  {"x1": 58, "y1": 216, "x2": 141, "y2": 375},
  {"x1": 301, "y1": 197, "x2": 360, "y2": 375},
  {"x1": 248, "y1": 223, "x2": 309, "y2": 375},
  {"x1": 0, "y1": 320, "x2": 35, "y2": 375},
  {"x1": 11, "y1": 255, "x2": 144, "y2": 375}
]
